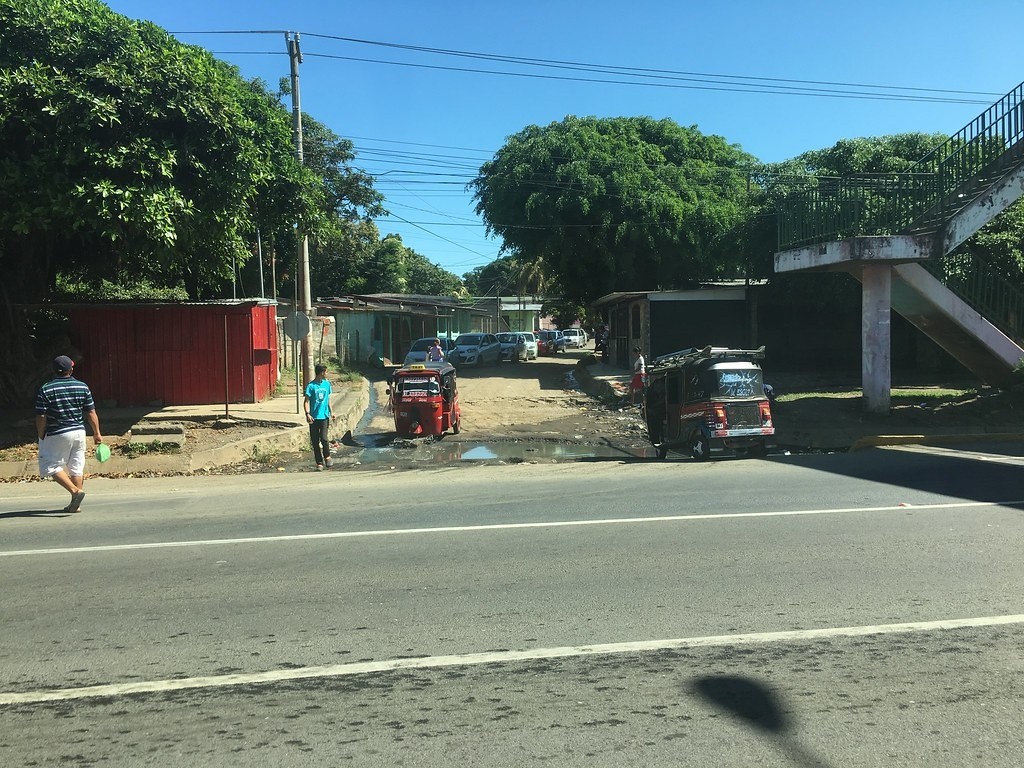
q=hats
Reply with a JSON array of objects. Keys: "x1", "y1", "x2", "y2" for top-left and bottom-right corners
[{"x1": 52, "y1": 355, "x2": 74, "y2": 372}]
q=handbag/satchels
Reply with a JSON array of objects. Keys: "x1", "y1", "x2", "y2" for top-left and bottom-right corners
[{"x1": 95, "y1": 443, "x2": 110, "y2": 463}]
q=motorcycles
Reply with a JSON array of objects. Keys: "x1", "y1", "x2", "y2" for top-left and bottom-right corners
[
  {"x1": 645, "y1": 344, "x2": 776, "y2": 464},
  {"x1": 388, "y1": 359, "x2": 461, "y2": 441}
]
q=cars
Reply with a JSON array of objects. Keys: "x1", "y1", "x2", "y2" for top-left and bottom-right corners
[
  {"x1": 403, "y1": 336, "x2": 460, "y2": 371},
  {"x1": 493, "y1": 333, "x2": 528, "y2": 365},
  {"x1": 577, "y1": 329, "x2": 590, "y2": 344},
  {"x1": 561, "y1": 328, "x2": 585, "y2": 349},
  {"x1": 527, "y1": 329, "x2": 553, "y2": 357},
  {"x1": 453, "y1": 333, "x2": 503, "y2": 372},
  {"x1": 513, "y1": 332, "x2": 538, "y2": 360},
  {"x1": 545, "y1": 329, "x2": 566, "y2": 356}
]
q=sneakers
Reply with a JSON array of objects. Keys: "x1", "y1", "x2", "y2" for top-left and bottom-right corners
[{"x1": 63, "y1": 489, "x2": 84, "y2": 513}]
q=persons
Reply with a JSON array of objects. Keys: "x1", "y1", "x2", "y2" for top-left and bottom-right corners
[
  {"x1": 428, "y1": 337, "x2": 444, "y2": 362},
  {"x1": 602, "y1": 325, "x2": 609, "y2": 358},
  {"x1": 594, "y1": 328, "x2": 602, "y2": 353},
  {"x1": 35, "y1": 356, "x2": 102, "y2": 513},
  {"x1": 304, "y1": 363, "x2": 336, "y2": 472},
  {"x1": 627, "y1": 346, "x2": 647, "y2": 403}
]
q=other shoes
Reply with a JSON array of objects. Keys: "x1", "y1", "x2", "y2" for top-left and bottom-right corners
[
  {"x1": 324, "y1": 456, "x2": 333, "y2": 467},
  {"x1": 316, "y1": 464, "x2": 322, "y2": 471}
]
